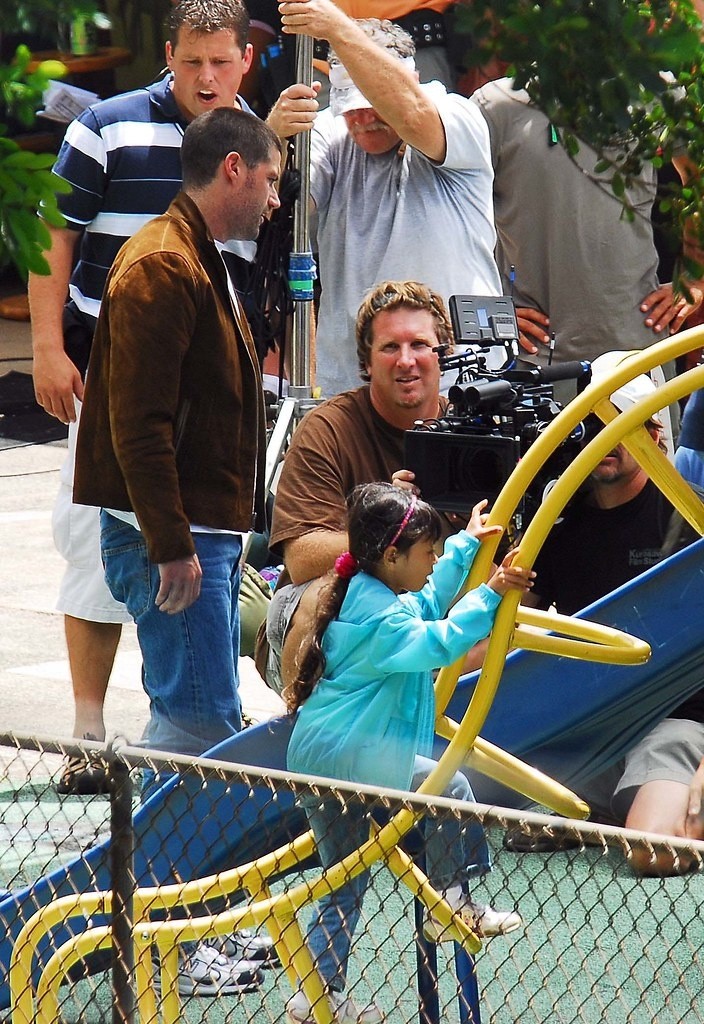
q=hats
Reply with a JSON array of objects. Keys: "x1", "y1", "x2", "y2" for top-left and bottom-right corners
[
  {"x1": 327, "y1": 57, "x2": 416, "y2": 118},
  {"x1": 580, "y1": 349, "x2": 667, "y2": 431}
]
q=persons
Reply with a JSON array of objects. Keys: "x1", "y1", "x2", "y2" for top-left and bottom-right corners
[
  {"x1": 70, "y1": 106, "x2": 286, "y2": 996},
  {"x1": 285, "y1": 484, "x2": 535, "y2": 1023},
  {"x1": 28, "y1": 0, "x2": 704, "y2": 878}
]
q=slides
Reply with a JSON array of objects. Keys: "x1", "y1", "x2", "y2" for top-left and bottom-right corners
[{"x1": 0, "y1": 534, "x2": 704, "y2": 1010}]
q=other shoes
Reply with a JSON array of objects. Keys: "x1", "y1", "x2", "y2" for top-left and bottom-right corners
[{"x1": 56, "y1": 756, "x2": 110, "y2": 798}]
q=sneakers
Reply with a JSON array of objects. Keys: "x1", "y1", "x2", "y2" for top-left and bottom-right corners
[
  {"x1": 151, "y1": 947, "x2": 266, "y2": 999},
  {"x1": 421, "y1": 902, "x2": 523, "y2": 944},
  {"x1": 202, "y1": 930, "x2": 280, "y2": 969},
  {"x1": 287, "y1": 1000, "x2": 383, "y2": 1024}
]
